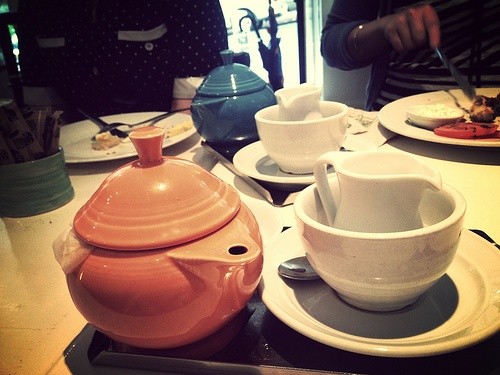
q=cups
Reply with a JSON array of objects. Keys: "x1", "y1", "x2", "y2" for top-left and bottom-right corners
[{"x1": 0, "y1": 146, "x2": 74, "y2": 218}]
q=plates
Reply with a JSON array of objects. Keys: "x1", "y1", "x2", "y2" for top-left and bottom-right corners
[
  {"x1": 258, "y1": 227, "x2": 500, "y2": 357},
  {"x1": 233, "y1": 133, "x2": 376, "y2": 190},
  {"x1": 406, "y1": 105, "x2": 464, "y2": 129},
  {"x1": 378, "y1": 88, "x2": 500, "y2": 147},
  {"x1": 59, "y1": 112, "x2": 197, "y2": 163}
]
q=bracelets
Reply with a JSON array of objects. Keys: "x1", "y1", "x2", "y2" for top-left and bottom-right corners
[{"x1": 352, "y1": 25, "x2": 372, "y2": 58}]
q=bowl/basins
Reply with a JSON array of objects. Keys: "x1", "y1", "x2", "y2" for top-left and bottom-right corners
[
  {"x1": 295, "y1": 176, "x2": 466, "y2": 312},
  {"x1": 254, "y1": 101, "x2": 349, "y2": 174}
]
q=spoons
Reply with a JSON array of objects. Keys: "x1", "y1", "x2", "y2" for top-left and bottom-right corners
[{"x1": 278, "y1": 256, "x2": 320, "y2": 281}]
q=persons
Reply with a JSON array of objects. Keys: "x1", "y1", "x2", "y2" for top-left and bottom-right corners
[
  {"x1": 321, "y1": 0, "x2": 500, "y2": 111},
  {"x1": 16, "y1": 0, "x2": 228, "y2": 122}
]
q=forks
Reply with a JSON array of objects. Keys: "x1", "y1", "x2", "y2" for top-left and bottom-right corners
[{"x1": 92, "y1": 108, "x2": 190, "y2": 140}]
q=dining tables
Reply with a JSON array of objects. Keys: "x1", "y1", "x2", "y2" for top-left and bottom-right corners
[{"x1": 0, "y1": 108, "x2": 500, "y2": 375}]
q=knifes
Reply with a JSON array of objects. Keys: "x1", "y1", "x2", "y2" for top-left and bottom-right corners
[
  {"x1": 436, "y1": 47, "x2": 476, "y2": 100},
  {"x1": 76, "y1": 108, "x2": 128, "y2": 139}
]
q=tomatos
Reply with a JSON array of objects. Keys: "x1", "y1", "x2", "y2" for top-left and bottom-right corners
[{"x1": 434, "y1": 121, "x2": 498, "y2": 139}]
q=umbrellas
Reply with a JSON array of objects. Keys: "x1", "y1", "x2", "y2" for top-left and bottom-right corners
[
  {"x1": 266, "y1": 0, "x2": 284, "y2": 85},
  {"x1": 239, "y1": 16, "x2": 283, "y2": 92}
]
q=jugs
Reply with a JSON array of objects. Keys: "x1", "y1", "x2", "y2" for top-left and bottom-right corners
[
  {"x1": 274, "y1": 82, "x2": 323, "y2": 122},
  {"x1": 315, "y1": 148, "x2": 442, "y2": 233}
]
